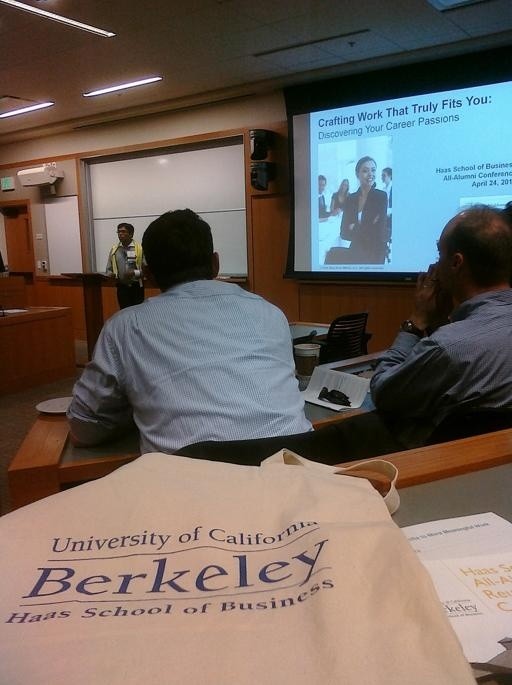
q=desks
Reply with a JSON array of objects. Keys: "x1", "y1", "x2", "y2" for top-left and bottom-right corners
[
  {"x1": 7, "y1": 349, "x2": 389, "y2": 509},
  {"x1": 330, "y1": 427, "x2": 512, "y2": 685},
  {"x1": 289, "y1": 321, "x2": 331, "y2": 346}
]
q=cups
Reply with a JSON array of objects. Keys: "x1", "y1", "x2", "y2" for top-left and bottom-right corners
[{"x1": 293, "y1": 344, "x2": 320, "y2": 378}]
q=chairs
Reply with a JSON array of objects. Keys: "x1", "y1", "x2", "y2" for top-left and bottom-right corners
[{"x1": 319, "y1": 312, "x2": 369, "y2": 365}]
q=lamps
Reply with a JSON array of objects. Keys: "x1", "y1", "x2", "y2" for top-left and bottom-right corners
[
  {"x1": 0, "y1": 101, "x2": 55, "y2": 118},
  {"x1": 0, "y1": 0, "x2": 117, "y2": 41},
  {"x1": 84, "y1": 77, "x2": 162, "y2": 98}
]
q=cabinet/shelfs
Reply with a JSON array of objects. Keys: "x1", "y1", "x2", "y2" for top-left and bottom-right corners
[{"x1": 0, "y1": 306, "x2": 78, "y2": 398}]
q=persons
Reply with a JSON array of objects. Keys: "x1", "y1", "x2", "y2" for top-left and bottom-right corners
[
  {"x1": 105, "y1": 221, "x2": 147, "y2": 306},
  {"x1": 318, "y1": 176, "x2": 330, "y2": 218},
  {"x1": 324, "y1": 156, "x2": 389, "y2": 263},
  {"x1": 378, "y1": 169, "x2": 392, "y2": 201},
  {"x1": 329, "y1": 180, "x2": 349, "y2": 214},
  {"x1": 68, "y1": 208, "x2": 317, "y2": 454},
  {"x1": 361, "y1": 206, "x2": 511, "y2": 454}
]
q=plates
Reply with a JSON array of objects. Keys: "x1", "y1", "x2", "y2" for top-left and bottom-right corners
[{"x1": 35, "y1": 397, "x2": 74, "y2": 413}]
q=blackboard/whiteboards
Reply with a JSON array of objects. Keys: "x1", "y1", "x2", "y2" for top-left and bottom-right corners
[
  {"x1": 42, "y1": 194, "x2": 82, "y2": 276},
  {"x1": 84, "y1": 143, "x2": 248, "y2": 279}
]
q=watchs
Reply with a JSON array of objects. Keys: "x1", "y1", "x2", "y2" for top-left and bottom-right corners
[{"x1": 400, "y1": 319, "x2": 425, "y2": 337}]
